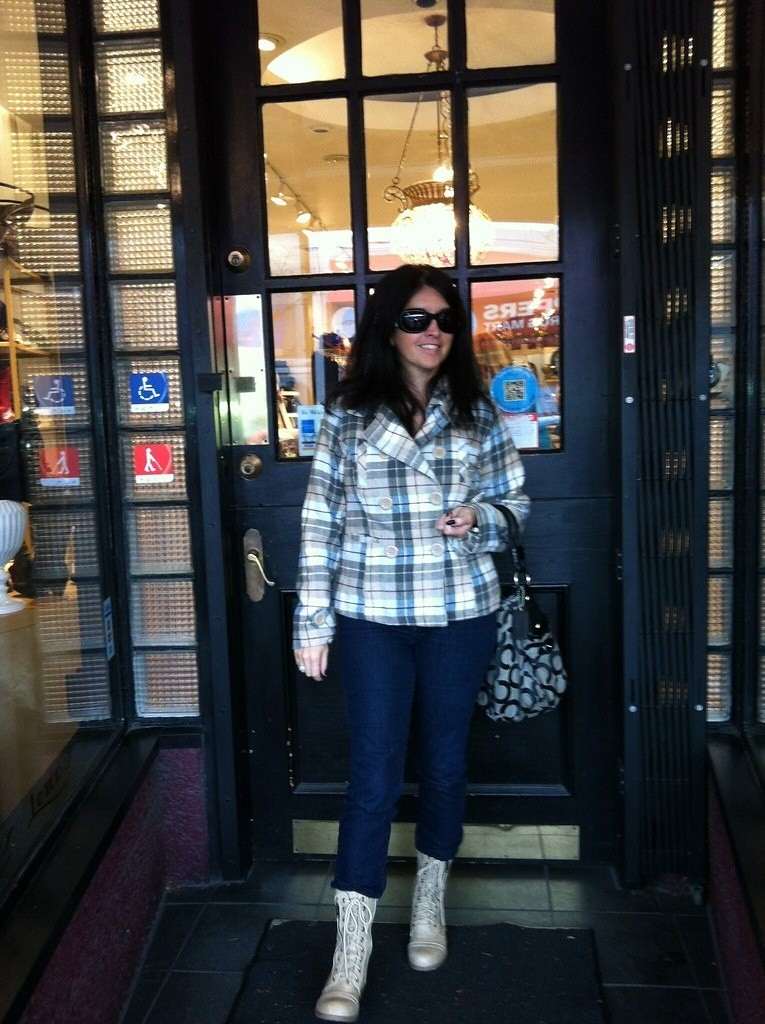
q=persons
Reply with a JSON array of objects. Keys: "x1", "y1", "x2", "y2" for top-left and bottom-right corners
[
  {"x1": 291, "y1": 262, "x2": 531, "y2": 1023},
  {"x1": 309, "y1": 331, "x2": 343, "y2": 406}
]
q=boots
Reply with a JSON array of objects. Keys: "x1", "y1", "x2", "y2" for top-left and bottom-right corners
[
  {"x1": 314, "y1": 887, "x2": 378, "y2": 1022},
  {"x1": 409, "y1": 847, "x2": 455, "y2": 972}
]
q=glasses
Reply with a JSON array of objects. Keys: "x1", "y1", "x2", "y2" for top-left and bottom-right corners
[{"x1": 394, "y1": 307, "x2": 457, "y2": 334}]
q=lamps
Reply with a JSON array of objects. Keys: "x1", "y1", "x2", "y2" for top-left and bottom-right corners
[{"x1": 384, "y1": 13, "x2": 493, "y2": 269}]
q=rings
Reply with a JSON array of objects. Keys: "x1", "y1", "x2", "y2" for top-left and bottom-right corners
[{"x1": 297, "y1": 664, "x2": 307, "y2": 673}]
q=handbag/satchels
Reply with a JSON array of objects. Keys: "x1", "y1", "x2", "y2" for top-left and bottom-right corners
[{"x1": 477, "y1": 504, "x2": 568, "y2": 726}]
